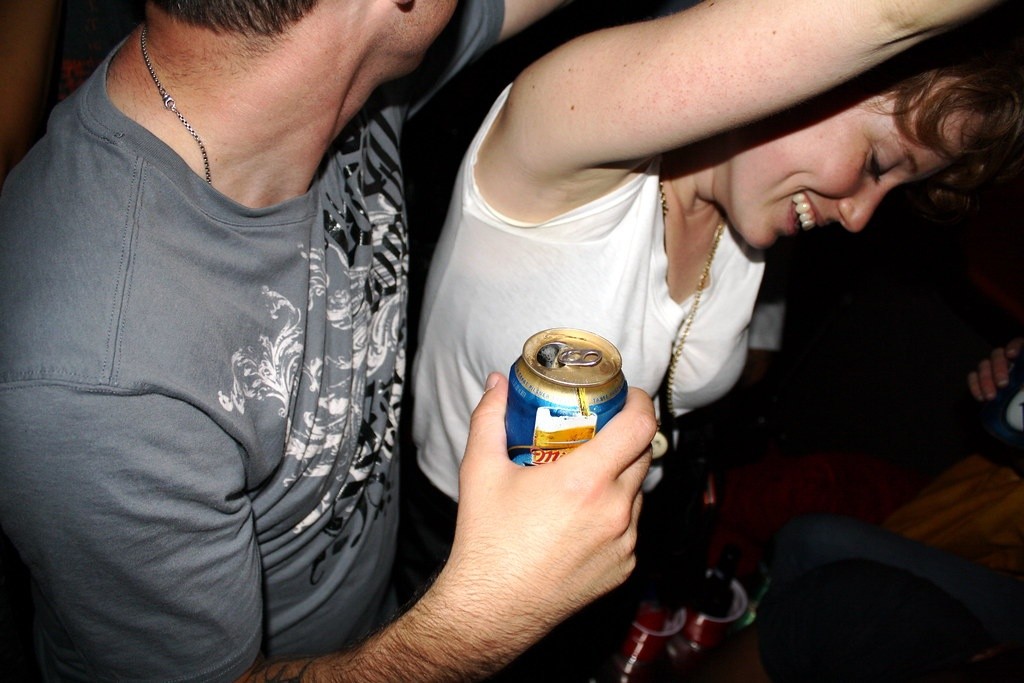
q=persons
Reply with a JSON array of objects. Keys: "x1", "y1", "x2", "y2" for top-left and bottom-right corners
[
  {"x1": 0, "y1": 0, "x2": 657, "y2": 683},
  {"x1": 411, "y1": 0, "x2": 1024, "y2": 683},
  {"x1": 774, "y1": 336, "x2": 1024, "y2": 636}
]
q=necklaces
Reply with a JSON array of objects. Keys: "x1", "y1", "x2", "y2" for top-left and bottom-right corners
[
  {"x1": 648, "y1": 159, "x2": 727, "y2": 460},
  {"x1": 141, "y1": 23, "x2": 212, "y2": 186}
]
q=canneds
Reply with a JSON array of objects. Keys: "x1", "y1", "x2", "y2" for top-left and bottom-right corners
[
  {"x1": 978, "y1": 341, "x2": 1023, "y2": 450},
  {"x1": 505, "y1": 329, "x2": 628, "y2": 468}
]
